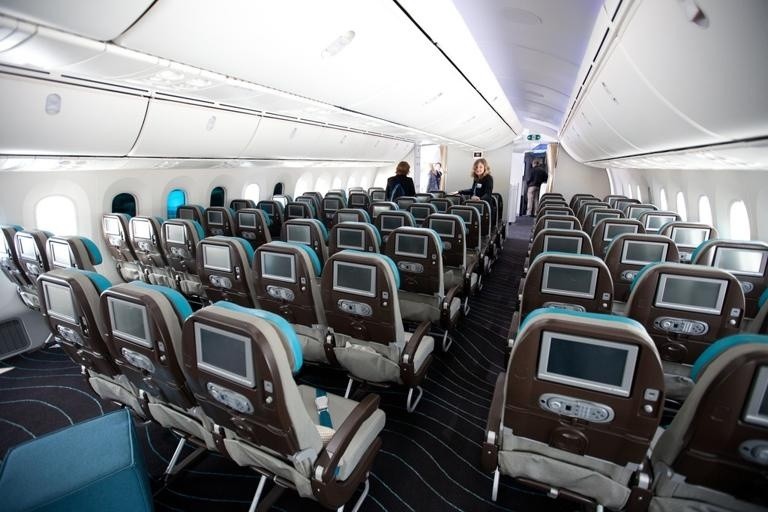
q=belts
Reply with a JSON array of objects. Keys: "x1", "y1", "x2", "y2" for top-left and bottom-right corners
[{"x1": 522, "y1": 214, "x2": 531, "y2": 217}]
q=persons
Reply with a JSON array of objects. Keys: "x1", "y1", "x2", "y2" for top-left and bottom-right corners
[
  {"x1": 522, "y1": 159, "x2": 547, "y2": 218},
  {"x1": 384, "y1": 161, "x2": 415, "y2": 201},
  {"x1": 427, "y1": 161, "x2": 442, "y2": 191},
  {"x1": 448, "y1": 157, "x2": 494, "y2": 202}
]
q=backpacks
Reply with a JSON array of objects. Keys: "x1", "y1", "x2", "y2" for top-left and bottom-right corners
[
  {"x1": 389, "y1": 181, "x2": 406, "y2": 201},
  {"x1": 537, "y1": 169, "x2": 548, "y2": 183}
]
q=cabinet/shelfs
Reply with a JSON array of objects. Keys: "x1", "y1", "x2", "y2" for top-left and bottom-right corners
[{"x1": 559, "y1": 0, "x2": 767, "y2": 171}]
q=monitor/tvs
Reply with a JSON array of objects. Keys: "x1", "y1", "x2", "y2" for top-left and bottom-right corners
[
  {"x1": 713, "y1": 247, "x2": 768, "y2": 277},
  {"x1": 194, "y1": 322, "x2": 256, "y2": 388},
  {"x1": 671, "y1": 227, "x2": 710, "y2": 248},
  {"x1": 541, "y1": 262, "x2": 599, "y2": 300},
  {"x1": 654, "y1": 273, "x2": 728, "y2": 316},
  {"x1": 537, "y1": 330, "x2": 639, "y2": 397},
  {"x1": 743, "y1": 366, "x2": 768, "y2": 427},
  {"x1": 42, "y1": 280, "x2": 79, "y2": 324},
  {"x1": 543, "y1": 196, "x2": 676, "y2": 265},
  {"x1": 106, "y1": 296, "x2": 153, "y2": 348}
]
